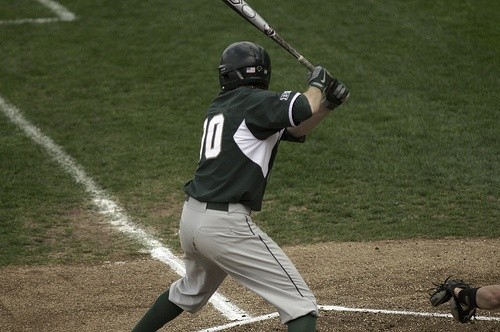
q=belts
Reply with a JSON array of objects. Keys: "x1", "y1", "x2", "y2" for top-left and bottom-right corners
[{"x1": 186, "y1": 195, "x2": 229, "y2": 211}]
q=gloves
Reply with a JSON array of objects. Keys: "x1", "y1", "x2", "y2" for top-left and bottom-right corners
[
  {"x1": 307, "y1": 65, "x2": 332, "y2": 92},
  {"x1": 326, "y1": 78, "x2": 349, "y2": 108}
]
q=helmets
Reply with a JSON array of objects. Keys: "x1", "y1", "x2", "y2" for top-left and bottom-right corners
[{"x1": 218, "y1": 41, "x2": 271, "y2": 91}]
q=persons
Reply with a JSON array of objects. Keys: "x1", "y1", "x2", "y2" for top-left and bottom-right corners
[
  {"x1": 127, "y1": 41, "x2": 347, "y2": 332},
  {"x1": 450, "y1": 281, "x2": 500, "y2": 323}
]
